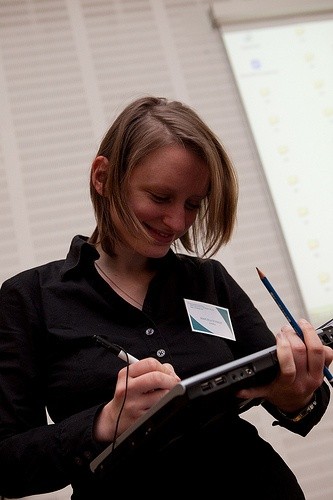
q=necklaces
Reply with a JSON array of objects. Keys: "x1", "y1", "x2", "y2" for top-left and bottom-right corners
[{"x1": 95, "y1": 257, "x2": 143, "y2": 307}]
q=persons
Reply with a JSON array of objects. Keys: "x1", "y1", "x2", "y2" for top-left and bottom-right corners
[{"x1": 0, "y1": 99, "x2": 333, "y2": 500}]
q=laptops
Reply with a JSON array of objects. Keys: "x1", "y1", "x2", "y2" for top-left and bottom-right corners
[{"x1": 89, "y1": 325, "x2": 333, "y2": 479}]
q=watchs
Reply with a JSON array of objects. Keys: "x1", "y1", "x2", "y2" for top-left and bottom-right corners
[{"x1": 276, "y1": 393, "x2": 319, "y2": 423}]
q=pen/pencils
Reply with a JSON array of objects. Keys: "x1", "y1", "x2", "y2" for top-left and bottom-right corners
[
  {"x1": 255, "y1": 267, "x2": 333, "y2": 389},
  {"x1": 94, "y1": 334, "x2": 140, "y2": 365}
]
